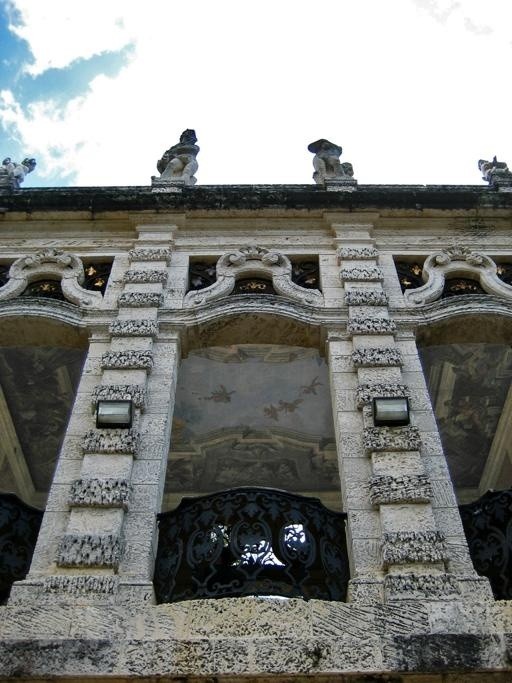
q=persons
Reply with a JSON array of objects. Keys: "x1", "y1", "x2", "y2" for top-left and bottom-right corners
[
  {"x1": 478, "y1": 156, "x2": 511, "y2": 180},
  {"x1": 7, "y1": 157, "x2": 37, "y2": 183},
  {"x1": 155, "y1": 128, "x2": 200, "y2": 185},
  {"x1": 307, "y1": 138, "x2": 343, "y2": 180}
]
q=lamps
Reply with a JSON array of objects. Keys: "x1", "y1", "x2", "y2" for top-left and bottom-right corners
[
  {"x1": 371, "y1": 396, "x2": 411, "y2": 427},
  {"x1": 96, "y1": 399, "x2": 135, "y2": 429}
]
